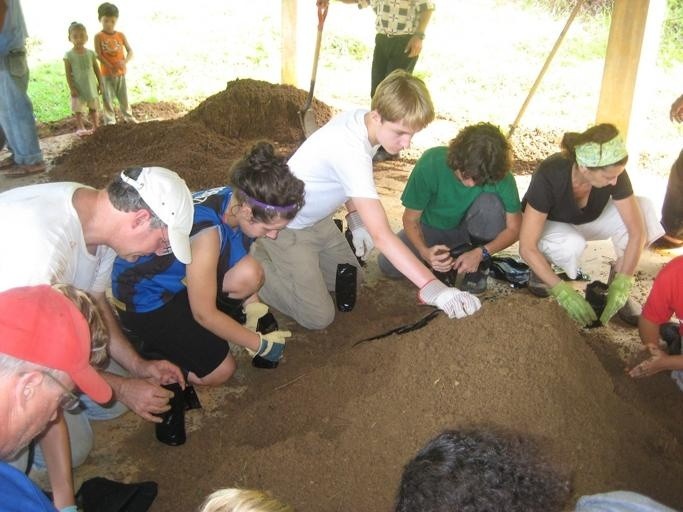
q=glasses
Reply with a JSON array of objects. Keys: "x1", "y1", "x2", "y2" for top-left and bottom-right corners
[
  {"x1": 34, "y1": 367, "x2": 81, "y2": 411},
  {"x1": 151, "y1": 223, "x2": 174, "y2": 261}
]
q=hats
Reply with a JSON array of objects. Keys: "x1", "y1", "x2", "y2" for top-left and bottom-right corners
[
  {"x1": 118, "y1": 164, "x2": 195, "y2": 263},
  {"x1": 0, "y1": 283, "x2": 113, "y2": 405}
]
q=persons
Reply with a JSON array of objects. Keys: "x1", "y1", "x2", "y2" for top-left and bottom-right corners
[
  {"x1": 397, "y1": 423, "x2": 682, "y2": 512},
  {"x1": 376, "y1": 123, "x2": 523, "y2": 294},
  {"x1": 1, "y1": 0, "x2": 48, "y2": 179},
  {"x1": 342, "y1": 1, "x2": 434, "y2": 161},
  {"x1": 0, "y1": 164, "x2": 194, "y2": 473},
  {"x1": 517, "y1": 123, "x2": 665, "y2": 329},
  {"x1": 0, "y1": 285, "x2": 113, "y2": 511},
  {"x1": 65, "y1": 22, "x2": 104, "y2": 136},
  {"x1": 249, "y1": 70, "x2": 481, "y2": 346},
  {"x1": 120, "y1": 143, "x2": 305, "y2": 447},
  {"x1": 653, "y1": 95, "x2": 682, "y2": 250},
  {"x1": 628, "y1": 256, "x2": 683, "y2": 391},
  {"x1": 96, "y1": 3, "x2": 133, "y2": 127}
]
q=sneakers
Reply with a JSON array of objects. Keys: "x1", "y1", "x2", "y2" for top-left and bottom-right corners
[
  {"x1": 651, "y1": 234, "x2": 683, "y2": 255},
  {"x1": 463, "y1": 264, "x2": 491, "y2": 292},
  {"x1": 434, "y1": 259, "x2": 457, "y2": 288}
]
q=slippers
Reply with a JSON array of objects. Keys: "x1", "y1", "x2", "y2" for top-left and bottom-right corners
[
  {"x1": 3, "y1": 166, "x2": 46, "y2": 181},
  {"x1": 0, "y1": 156, "x2": 17, "y2": 169}
]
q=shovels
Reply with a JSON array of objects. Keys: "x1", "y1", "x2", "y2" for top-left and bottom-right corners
[{"x1": 297, "y1": 4, "x2": 329, "y2": 137}]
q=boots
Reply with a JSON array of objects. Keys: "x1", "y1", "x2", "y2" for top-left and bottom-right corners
[
  {"x1": 526, "y1": 262, "x2": 551, "y2": 299},
  {"x1": 607, "y1": 258, "x2": 647, "y2": 328}
]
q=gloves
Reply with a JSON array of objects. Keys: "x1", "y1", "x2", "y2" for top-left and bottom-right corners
[
  {"x1": 417, "y1": 278, "x2": 481, "y2": 319},
  {"x1": 345, "y1": 210, "x2": 375, "y2": 261},
  {"x1": 245, "y1": 301, "x2": 278, "y2": 333},
  {"x1": 544, "y1": 280, "x2": 598, "y2": 328},
  {"x1": 598, "y1": 260, "x2": 642, "y2": 326},
  {"x1": 244, "y1": 329, "x2": 292, "y2": 362}
]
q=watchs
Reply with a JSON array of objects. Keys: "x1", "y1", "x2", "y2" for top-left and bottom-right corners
[
  {"x1": 480, "y1": 246, "x2": 493, "y2": 261},
  {"x1": 412, "y1": 31, "x2": 424, "y2": 38}
]
